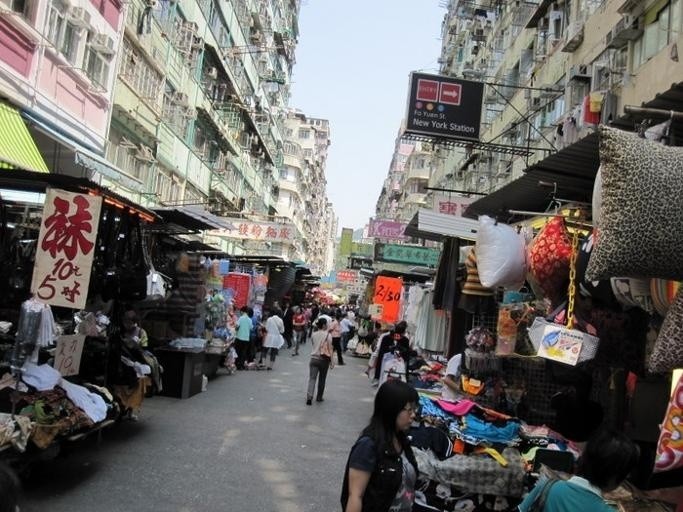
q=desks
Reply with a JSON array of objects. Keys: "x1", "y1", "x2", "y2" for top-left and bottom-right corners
[
  {"x1": 153, "y1": 344, "x2": 203, "y2": 399},
  {"x1": 69, "y1": 419, "x2": 117, "y2": 444}
]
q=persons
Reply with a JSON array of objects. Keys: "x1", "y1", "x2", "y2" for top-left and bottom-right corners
[
  {"x1": 120, "y1": 309, "x2": 149, "y2": 421},
  {"x1": 231, "y1": 300, "x2": 412, "y2": 376},
  {"x1": 516, "y1": 427, "x2": 642, "y2": 511},
  {"x1": 305, "y1": 318, "x2": 334, "y2": 404},
  {"x1": 442, "y1": 352, "x2": 462, "y2": 403},
  {"x1": 338, "y1": 381, "x2": 421, "y2": 511}
]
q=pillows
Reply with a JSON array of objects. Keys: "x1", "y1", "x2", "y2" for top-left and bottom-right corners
[
  {"x1": 610, "y1": 276, "x2": 653, "y2": 316},
  {"x1": 477, "y1": 214, "x2": 527, "y2": 292},
  {"x1": 647, "y1": 281, "x2": 682, "y2": 373},
  {"x1": 522, "y1": 211, "x2": 577, "y2": 298},
  {"x1": 585, "y1": 124, "x2": 683, "y2": 284},
  {"x1": 592, "y1": 117, "x2": 671, "y2": 230}
]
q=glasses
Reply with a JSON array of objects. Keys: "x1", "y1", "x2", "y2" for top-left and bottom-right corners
[{"x1": 403, "y1": 406, "x2": 419, "y2": 414}]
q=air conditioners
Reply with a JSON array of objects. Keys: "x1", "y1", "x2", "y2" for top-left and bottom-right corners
[
  {"x1": 494, "y1": 14, "x2": 642, "y2": 178},
  {"x1": 71, "y1": 6, "x2": 216, "y2": 160}
]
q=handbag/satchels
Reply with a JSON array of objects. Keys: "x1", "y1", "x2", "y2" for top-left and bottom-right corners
[
  {"x1": 356, "y1": 325, "x2": 369, "y2": 354},
  {"x1": 320, "y1": 340, "x2": 331, "y2": 358}
]
